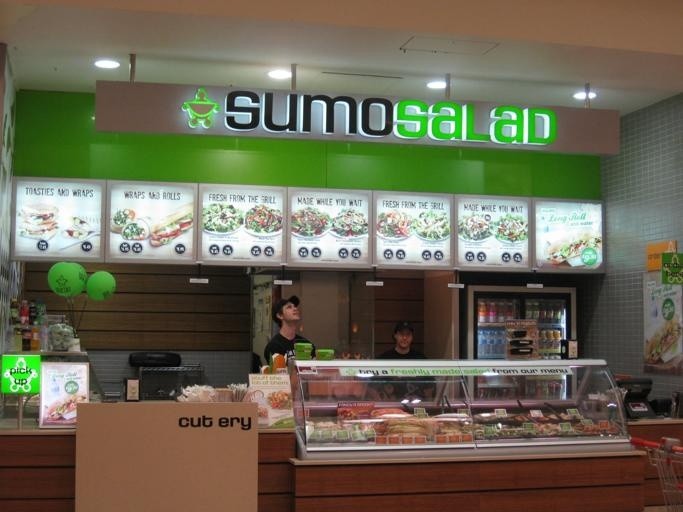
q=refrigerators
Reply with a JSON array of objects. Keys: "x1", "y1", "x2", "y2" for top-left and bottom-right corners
[{"x1": 425, "y1": 269, "x2": 578, "y2": 400}]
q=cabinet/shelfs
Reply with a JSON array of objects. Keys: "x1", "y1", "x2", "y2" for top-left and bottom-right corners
[
  {"x1": 283, "y1": 356, "x2": 633, "y2": 459},
  {"x1": 0, "y1": 349, "x2": 104, "y2": 430}
]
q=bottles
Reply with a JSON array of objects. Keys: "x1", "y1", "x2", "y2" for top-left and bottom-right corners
[
  {"x1": 475, "y1": 300, "x2": 562, "y2": 399},
  {"x1": 9, "y1": 296, "x2": 75, "y2": 352}
]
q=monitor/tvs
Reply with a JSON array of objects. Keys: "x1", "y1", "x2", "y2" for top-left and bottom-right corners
[{"x1": 617, "y1": 379, "x2": 653, "y2": 401}]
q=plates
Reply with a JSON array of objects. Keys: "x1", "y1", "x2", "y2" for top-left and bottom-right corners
[
  {"x1": 243, "y1": 226, "x2": 283, "y2": 236},
  {"x1": 203, "y1": 223, "x2": 245, "y2": 235},
  {"x1": 412, "y1": 227, "x2": 450, "y2": 242},
  {"x1": 458, "y1": 233, "x2": 494, "y2": 242},
  {"x1": 543, "y1": 242, "x2": 600, "y2": 267},
  {"x1": 290, "y1": 223, "x2": 331, "y2": 239},
  {"x1": 377, "y1": 228, "x2": 414, "y2": 241},
  {"x1": 328, "y1": 229, "x2": 367, "y2": 238},
  {"x1": 494, "y1": 234, "x2": 526, "y2": 244}
]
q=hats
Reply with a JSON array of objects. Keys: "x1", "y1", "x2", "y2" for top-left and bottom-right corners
[
  {"x1": 395, "y1": 320, "x2": 415, "y2": 331},
  {"x1": 270, "y1": 294, "x2": 299, "y2": 325}
]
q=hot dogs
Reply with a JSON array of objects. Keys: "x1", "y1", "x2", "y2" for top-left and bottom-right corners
[{"x1": 150, "y1": 203, "x2": 193, "y2": 247}]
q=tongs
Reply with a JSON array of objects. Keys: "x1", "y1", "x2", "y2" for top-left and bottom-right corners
[
  {"x1": 441, "y1": 395, "x2": 455, "y2": 414},
  {"x1": 543, "y1": 402, "x2": 567, "y2": 423}
]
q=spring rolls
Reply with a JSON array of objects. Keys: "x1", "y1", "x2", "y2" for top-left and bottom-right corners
[
  {"x1": 121, "y1": 215, "x2": 152, "y2": 241},
  {"x1": 111, "y1": 209, "x2": 135, "y2": 233}
]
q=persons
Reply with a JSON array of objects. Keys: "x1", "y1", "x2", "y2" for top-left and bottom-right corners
[
  {"x1": 376, "y1": 321, "x2": 426, "y2": 358},
  {"x1": 264, "y1": 295, "x2": 317, "y2": 364}
]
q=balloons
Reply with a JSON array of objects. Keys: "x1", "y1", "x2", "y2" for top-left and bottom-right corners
[
  {"x1": 70, "y1": 263, "x2": 87, "y2": 297},
  {"x1": 47, "y1": 262, "x2": 77, "y2": 298},
  {"x1": 86, "y1": 270, "x2": 115, "y2": 303}
]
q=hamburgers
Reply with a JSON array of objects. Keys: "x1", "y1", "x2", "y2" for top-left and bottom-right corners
[{"x1": 18, "y1": 203, "x2": 57, "y2": 240}]
